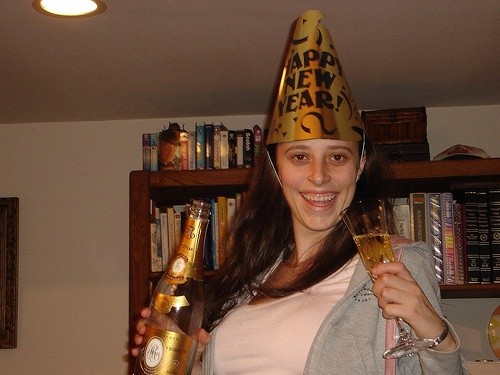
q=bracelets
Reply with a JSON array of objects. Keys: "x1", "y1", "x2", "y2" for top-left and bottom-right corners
[{"x1": 428, "y1": 320, "x2": 449, "y2": 348}]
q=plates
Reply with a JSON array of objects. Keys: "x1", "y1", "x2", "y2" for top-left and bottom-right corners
[{"x1": 487, "y1": 305, "x2": 500, "y2": 359}]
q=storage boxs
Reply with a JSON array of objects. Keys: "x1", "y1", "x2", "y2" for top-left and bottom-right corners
[{"x1": 361, "y1": 106, "x2": 431, "y2": 159}]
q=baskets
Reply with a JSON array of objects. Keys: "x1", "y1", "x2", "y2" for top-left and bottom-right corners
[{"x1": 361, "y1": 107, "x2": 428, "y2": 144}]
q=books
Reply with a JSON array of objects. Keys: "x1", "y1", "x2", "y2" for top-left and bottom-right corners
[
  {"x1": 150, "y1": 193, "x2": 244, "y2": 272},
  {"x1": 388, "y1": 191, "x2": 500, "y2": 284},
  {"x1": 142, "y1": 123, "x2": 261, "y2": 170}
]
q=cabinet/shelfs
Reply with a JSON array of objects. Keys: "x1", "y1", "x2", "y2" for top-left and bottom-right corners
[{"x1": 129, "y1": 159, "x2": 500, "y2": 375}]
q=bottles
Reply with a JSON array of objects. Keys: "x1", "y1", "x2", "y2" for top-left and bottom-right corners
[{"x1": 132, "y1": 199, "x2": 212, "y2": 375}]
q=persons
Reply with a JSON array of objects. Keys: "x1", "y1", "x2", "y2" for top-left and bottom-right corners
[{"x1": 132, "y1": 10, "x2": 463, "y2": 375}]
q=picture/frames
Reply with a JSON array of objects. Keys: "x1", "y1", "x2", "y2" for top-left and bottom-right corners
[{"x1": 0, "y1": 197, "x2": 20, "y2": 350}]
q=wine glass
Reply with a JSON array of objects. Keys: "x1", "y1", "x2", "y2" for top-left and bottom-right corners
[{"x1": 339, "y1": 199, "x2": 439, "y2": 360}]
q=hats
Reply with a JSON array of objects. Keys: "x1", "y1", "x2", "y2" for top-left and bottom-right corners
[{"x1": 264, "y1": 10, "x2": 364, "y2": 146}]
